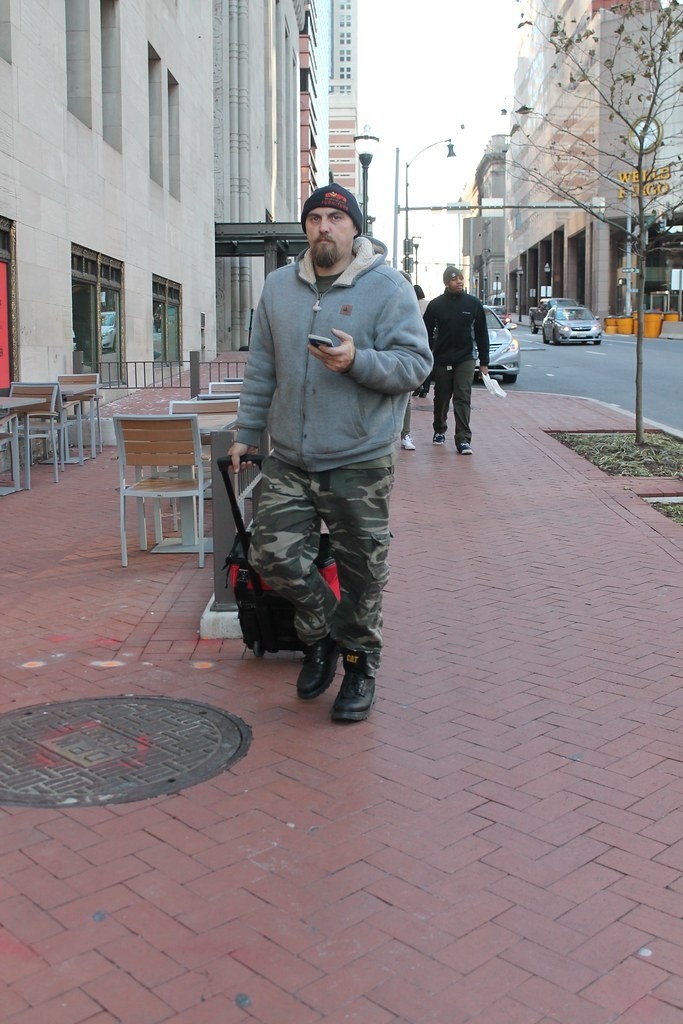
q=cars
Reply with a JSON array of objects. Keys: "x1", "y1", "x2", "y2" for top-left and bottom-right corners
[
  {"x1": 541, "y1": 305, "x2": 603, "y2": 346},
  {"x1": 473, "y1": 304, "x2": 521, "y2": 384},
  {"x1": 488, "y1": 305, "x2": 513, "y2": 331},
  {"x1": 101, "y1": 311, "x2": 116, "y2": 351}
]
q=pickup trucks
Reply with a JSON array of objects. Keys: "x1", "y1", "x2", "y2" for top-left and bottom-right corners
[{"x1": 529, "y1": 297, "x2": 582, "y2": 335}]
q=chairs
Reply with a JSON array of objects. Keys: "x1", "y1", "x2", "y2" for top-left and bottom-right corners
[
  {"x1": 112, "y1": 413, "x2": 211, "y2": 568},
  {"x1": 0, "y1": 413, "x2": 24, "y2": 497},
  {"x1": 169, "y1": 377, "x2": 244, "y2": 417},
  {"x1": 5, "y1": 384, "x2": 59, "y2": 490},
  {"x1": 57, "y1": 373, "x2": 102, "y2": 456},
  {"x1": 11, "y1": 382, "x2": 84, "y2": 472}
]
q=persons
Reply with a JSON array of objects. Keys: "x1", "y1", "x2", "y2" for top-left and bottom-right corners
[
  {"x1": 396, "y1": 269, "x2": 416, "y2": 451},
  {"x1": 228, "y1": 183, "x2": 433, "y2": 722},
  {"x1": 423, "y1": 266, "x2": 490, "y2": 455},
  {"x1": 411, "y1": 285, "x2": 432, "y2": 398}
]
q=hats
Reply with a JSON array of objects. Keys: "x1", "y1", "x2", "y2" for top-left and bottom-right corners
[
  {"x1": 399, "y1": 271, "x2": 412, "y2": 284},
  {"x1": 300, "y1": 183, "x2": 363, "y2": 240},
  {"x1": 443, "y1": 266, "x2": 464, "y2": 285}
]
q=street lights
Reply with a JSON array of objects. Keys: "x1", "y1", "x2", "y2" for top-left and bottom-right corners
[
  {"x1": 573, "y1": 169, "x2": 632, "y2": 316},
  {"x1": 495, "y1": 272, "x2": 500, "y2": 306},
  {"x1": 352, "y1": 126, "x2": 380, "y2": 235},
  {"x1": 544, "y1": 262, "x2": 550, "y2": 297},
  {"x1": 483, "y1": 274, "x2": 489, "y2": 304},
  {"x1": 405, "y1": 139, "x2": 456, "y2": 273},
  {"x1": 517, "y1": 267, "x2": 524, "y2": 322},
  {"x1": 411, "y1": 236, "x2": 422, "y2": 285}
]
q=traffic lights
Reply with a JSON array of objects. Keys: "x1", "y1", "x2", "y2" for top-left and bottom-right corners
[{"x1": 618, "y1": 278, "x2": 626, "y2": 285}]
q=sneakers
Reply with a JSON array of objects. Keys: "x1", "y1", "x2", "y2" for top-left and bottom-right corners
[
  {"x1": 401, "y1": 434, "x2": 415, "y2": 449},
  {"x1": 297, "y1": 632, "x2": 341, "y2": 699},
  {"x1": 331, "y1": 647, "x2": 376, "y2": 721},
  {"x1": 433, "y1": 432, "x2": 445, "y2": 445},
  {"x1": 457, "y1": 442, "x2": 473, "y2": 454}
]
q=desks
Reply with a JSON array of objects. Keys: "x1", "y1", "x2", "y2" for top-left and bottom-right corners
[
  {"x1": 60, "y1": 384, "x2": 103, "y2": 467},
  {"x1": 150, "y1": 415, "x2": 238, "y2": 553},
  {"x1": 0, "y1": 396, "x2": 46, "y2": 490}
]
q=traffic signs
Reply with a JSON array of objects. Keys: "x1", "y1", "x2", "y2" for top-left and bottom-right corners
[{"x1": 621, "y1": 267, "x2": 635, "y2": 273}]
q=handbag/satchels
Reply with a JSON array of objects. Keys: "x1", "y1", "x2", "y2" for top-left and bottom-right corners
[{"x1": 481, "y1": 372, "x2": 507, "y2": 398}]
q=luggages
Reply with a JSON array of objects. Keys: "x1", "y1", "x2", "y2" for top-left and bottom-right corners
[{"x1": 217, "y1": 454, "x2": 341, "y2": 658}]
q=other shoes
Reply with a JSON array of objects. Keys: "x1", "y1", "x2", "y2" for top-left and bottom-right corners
[
  {"x1": 419, "y1": 393, "x2": 427, "y2": 398},
  {"x1": 412, "y1": 384, "x2": 424, "y2": 396}
]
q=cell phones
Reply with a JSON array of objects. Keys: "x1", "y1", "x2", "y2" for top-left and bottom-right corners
[{"x1": 308, "y1": 334, "x2": 334, "y2": 349}]
